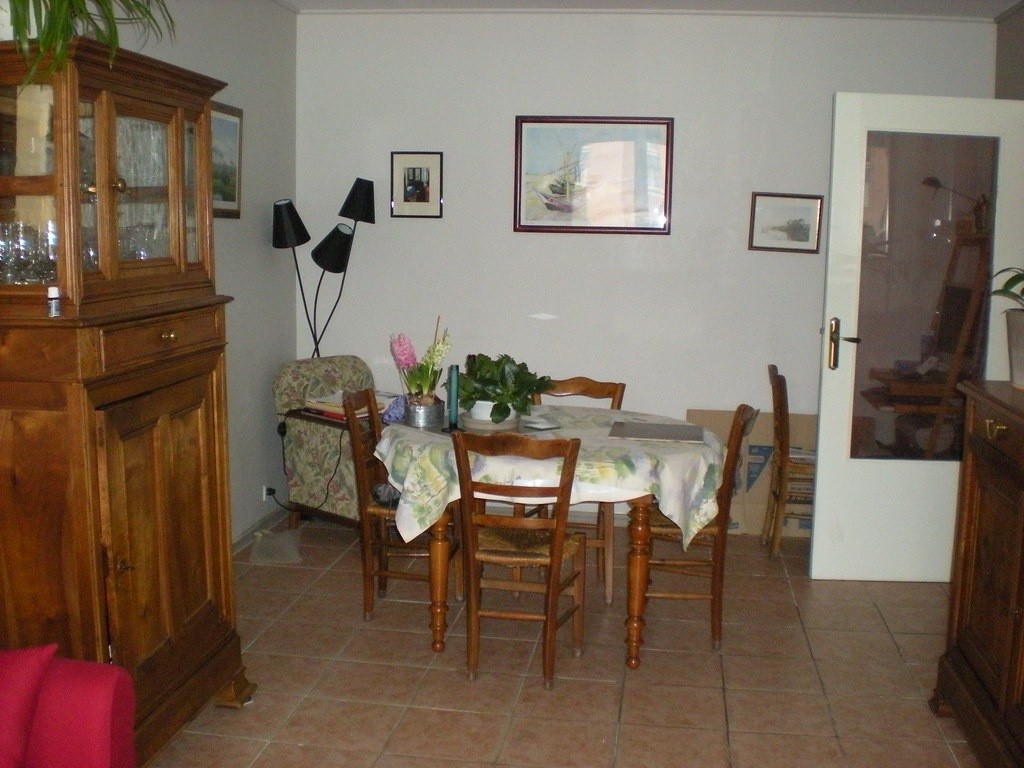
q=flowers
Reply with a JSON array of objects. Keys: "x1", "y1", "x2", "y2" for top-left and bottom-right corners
[{"x1": 390, "y1": 317, "x2": 453, "y2": 399}]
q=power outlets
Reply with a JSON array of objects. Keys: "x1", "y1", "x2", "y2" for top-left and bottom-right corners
[{"x1": 262, "y1": 484, "x2": 275, "y2": 500}]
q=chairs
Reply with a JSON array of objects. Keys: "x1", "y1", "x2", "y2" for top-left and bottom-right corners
[
  {"x1": 764, "y1": 364, "x2": 861, "y2": 556},
  {"x1": 510, "y1": 376, "x2": 627, "y2": 605},
  {"x1": 341, "y1": 388, "x2": 465, "y2": 653},
  {"x1": 465, "y1": 429, "x2": 584, "y2": 687},
  {"x1": 631, "y1": 402, "x2": 765, "y2": 653}
]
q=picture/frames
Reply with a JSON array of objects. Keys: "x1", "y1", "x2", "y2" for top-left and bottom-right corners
[
  {"x1": 204, "y1": 100, "x2": 245, "y2": 219},
  {"x1": 747, "y1": 190, "x2": 823, "y2": 254},
  {"x1": 390, "y1": 150, "x2": 445, "y2": 219},
  {"x1": 512, "y1": 116, "x2": 675, "y2": 234}
]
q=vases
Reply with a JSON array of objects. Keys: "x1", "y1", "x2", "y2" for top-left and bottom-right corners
[{"x1": 407, "y1": 389, "x2": 442, "y2": 424}]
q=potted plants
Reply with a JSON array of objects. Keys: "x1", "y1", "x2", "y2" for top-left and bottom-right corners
[
  {"x1": 439, "y1": 355, "x2": 550, "y2": 421},
  {"x1": 991, "y1": 264, "x2": 1024, "y2": 391}
]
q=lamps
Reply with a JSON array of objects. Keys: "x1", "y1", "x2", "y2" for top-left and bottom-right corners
[
  {"x1": 274, "y1": 177, "x2": 378, "y2": 362},
  {"x1": 922, "y1": 175, "x2": 984, "y2": 204}
]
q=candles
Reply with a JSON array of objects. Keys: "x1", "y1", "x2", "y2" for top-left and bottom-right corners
[{"x1": 446, "y1": 364, "x2": 458, "y2": 423}]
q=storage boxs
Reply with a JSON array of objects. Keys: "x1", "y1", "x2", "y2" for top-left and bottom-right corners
[{"x1": 685, "y1": 408, "x2": 875, "y2": 541}]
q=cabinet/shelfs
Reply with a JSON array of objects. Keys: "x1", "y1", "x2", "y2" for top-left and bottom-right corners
[
  {"x1": 1, "y1": 34, "x2": 256, "y2": 764},
  {"x1": 926, "y1": 379, "x2": 1024, "y2": 768}
]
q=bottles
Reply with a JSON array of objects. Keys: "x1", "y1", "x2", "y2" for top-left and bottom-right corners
[{"x1": 46, "y1": 289, "x2": 60, "y2": 319}]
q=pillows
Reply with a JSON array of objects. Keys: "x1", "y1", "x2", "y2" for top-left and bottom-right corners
[{"x1": 0, "y1": 644, "x2": 60, "y2": 768}]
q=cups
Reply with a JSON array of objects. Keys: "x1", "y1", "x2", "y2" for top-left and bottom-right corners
[{"x1": 0, "y1": 221, "x2": 176, "y2": 286}]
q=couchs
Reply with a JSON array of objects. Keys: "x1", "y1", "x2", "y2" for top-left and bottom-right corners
[
  {"x1": 274, "y1": 354, "x2": 389, "y2": 518},
  {"x1": 0, "y1": 643, "x2": 136, "y2": 768}
]
